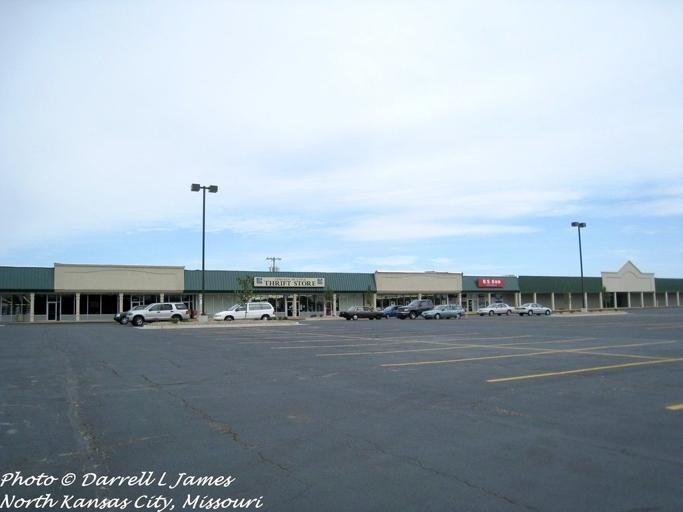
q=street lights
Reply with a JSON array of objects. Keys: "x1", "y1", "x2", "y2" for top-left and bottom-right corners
[
  {"x1": 571, "y1": 222, "x2": 587, "y2": 308},
  {"x1": 190, "y1": 184, "x2": 218, "y2": 316},
  {"x1": 213, "y1": 301, "x2": 276, "y2": 321}
]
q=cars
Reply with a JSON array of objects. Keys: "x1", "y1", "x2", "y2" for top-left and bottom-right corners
[
  {"x1": 113, "y1": 302, "x2": 190, "y2": 326},
  {"x1": 476, "y1": 303, "x2": 553, "y2": 316},
  {"x1": 339, "y1": 300, "x2": 465, "y2": 320}
]
